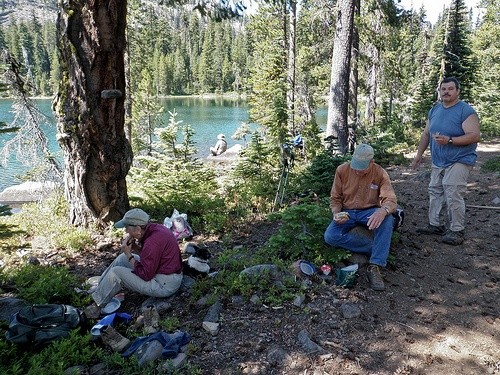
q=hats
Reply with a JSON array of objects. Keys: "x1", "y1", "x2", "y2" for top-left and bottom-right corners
[
  {"x1": 350, "y1": 144, "x2": 374, "y2": 171},
  {"x1": 114, "y1": 209, "x2": 150, "y2": 228},
  {"x1": 217, "y1": 133, "x2": 225, "y2": 140},
  {"x1": 172, "y1": 217, "x2": 189, "y2": 239}
]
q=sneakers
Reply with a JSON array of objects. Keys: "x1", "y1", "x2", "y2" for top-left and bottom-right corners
[
  {"x1": 288, "y1": 260, "x2": 323, "y2": 284},
  {"x1": 99, "y1": 325, "x2": 130, "y2": 353},
  {"x1": 140, "y1": 304, "x2": 160, "y2": 336},
  {"x1": 365, "y1": 265, "x2": 385, "y2": 291}
]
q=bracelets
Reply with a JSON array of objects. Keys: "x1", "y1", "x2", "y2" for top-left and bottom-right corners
[
  {"x1": 383, "y1": 206, "x2": 391, "y2": 214},
  {"x1": 127, "y1": 256, "x2": 134, "y2": 262}
]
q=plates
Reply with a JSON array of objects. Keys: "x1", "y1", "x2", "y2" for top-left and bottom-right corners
[{"x1": 101, "y1": 297, "x2": 121, "y2": 314}]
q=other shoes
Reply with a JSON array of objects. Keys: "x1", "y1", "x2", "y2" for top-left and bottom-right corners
[
  {"x1": 441, "y1": 228, "x2": 467, "y2": 246},
  {"x1": 415, "y1": 224, "x2": 447, "y2": 236}
]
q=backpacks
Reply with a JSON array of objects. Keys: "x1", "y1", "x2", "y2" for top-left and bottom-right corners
[{"x1": 6, "y1": 303, "x2": 81, "y2": 344}]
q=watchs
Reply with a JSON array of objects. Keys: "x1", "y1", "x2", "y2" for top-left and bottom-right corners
[{"x1": 448, "y1": 136, "x2": 453, "y2": 145}]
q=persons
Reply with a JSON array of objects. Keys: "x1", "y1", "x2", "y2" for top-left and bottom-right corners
[
  {"x1": 209, "y1": 133, "x2": 228, "y2": 157},
  {"x1": 81, "y1": 208, "x2": 185, "y2": 319},
  {"x1": 410, "y1": 76, "x2": 481, "y2": 247},
  {"x1": 323, "y1": 143, "x2": 398, "y2": 291}
]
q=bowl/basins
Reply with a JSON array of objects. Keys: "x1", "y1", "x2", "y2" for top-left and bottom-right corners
[{"x1": 336, "y1": 269, "x2": 358, "y2": 290}]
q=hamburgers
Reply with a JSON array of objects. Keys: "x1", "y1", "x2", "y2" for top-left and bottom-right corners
[
  {"x1": 121, "y1": 235, "x2": 135, "y2": 245},
  {"x1": 335, "y1": 212, "x2": 351, "y2": 221}
]
